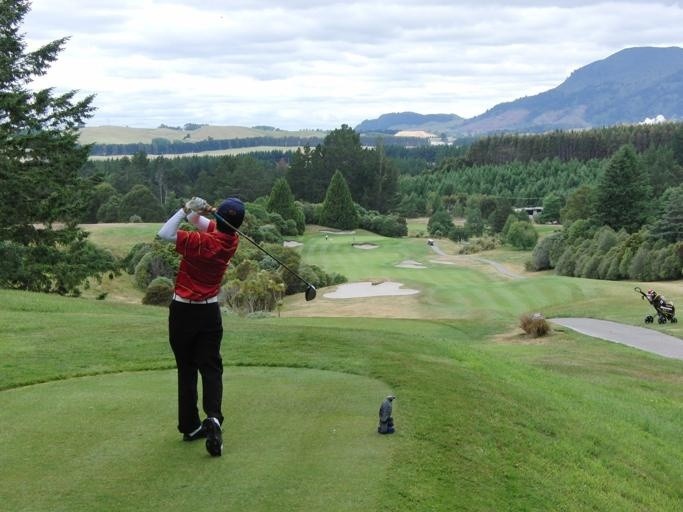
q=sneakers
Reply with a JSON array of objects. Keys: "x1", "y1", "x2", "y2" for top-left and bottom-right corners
[
  {"x1": 204, "y1": 417, "x2": 223, "y2": 456},
  {"x1": 183, "y1": 426, "x2": 207, "y2": 440}
]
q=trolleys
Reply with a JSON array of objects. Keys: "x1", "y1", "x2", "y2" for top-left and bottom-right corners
[{"x1": 635, "y1": 286, "x2": 678, "y2": 325}]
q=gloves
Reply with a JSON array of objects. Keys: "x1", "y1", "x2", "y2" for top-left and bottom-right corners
[{"x1": 186, "y1": 197, "x2": 206, "y2": 212}]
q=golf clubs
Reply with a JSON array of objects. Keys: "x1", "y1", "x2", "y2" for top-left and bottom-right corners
[{"x1": 204, "y1": 204, "x2": 316, "y2": 301}]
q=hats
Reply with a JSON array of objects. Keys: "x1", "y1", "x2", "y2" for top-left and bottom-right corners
[{"x1": 218, "y1": 198, "x2": 245, "y2": 217}]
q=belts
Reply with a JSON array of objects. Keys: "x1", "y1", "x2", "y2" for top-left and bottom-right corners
[{"x1": 172, "y1": 293, "x2": 217, "y2": 304}]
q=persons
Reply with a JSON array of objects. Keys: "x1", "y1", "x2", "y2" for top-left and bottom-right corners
[{"x1": 157, "y1": 195, "x2": 245, "y2": 457}]
